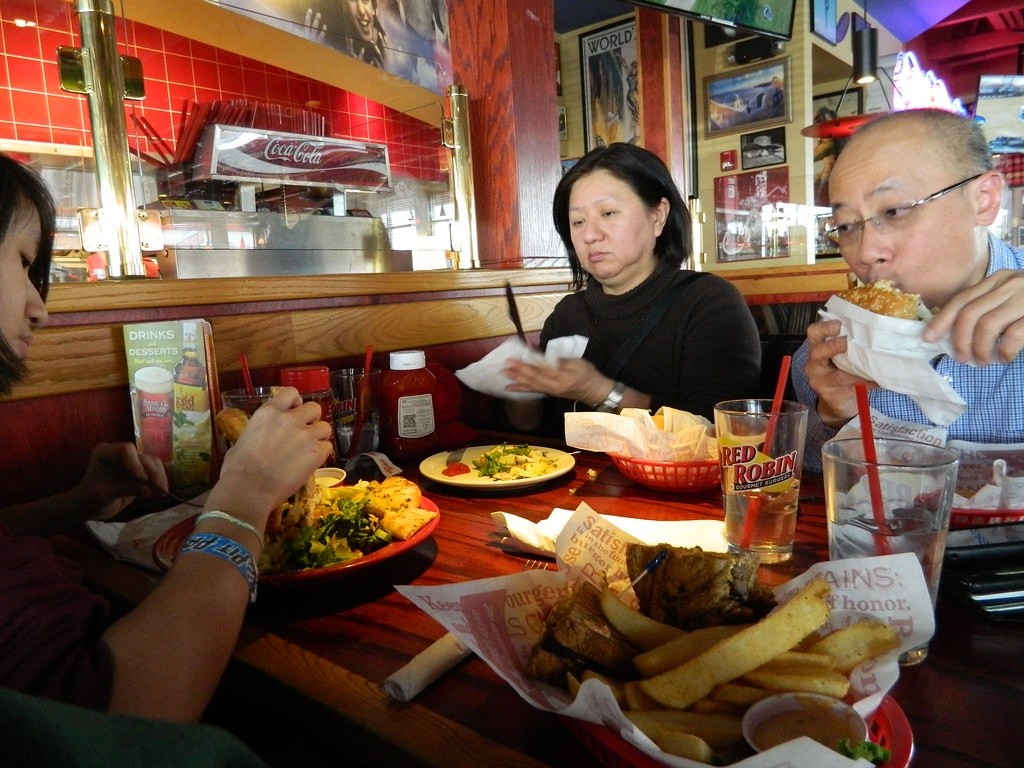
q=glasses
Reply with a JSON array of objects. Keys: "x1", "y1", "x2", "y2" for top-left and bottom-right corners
[{"x1": 824, "y1": 172, "x2": 984, "y2": 248}]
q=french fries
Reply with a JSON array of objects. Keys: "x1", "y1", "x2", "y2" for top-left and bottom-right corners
[{"x1": 565, "y1": 583, "x2": 903, "y2": 766}]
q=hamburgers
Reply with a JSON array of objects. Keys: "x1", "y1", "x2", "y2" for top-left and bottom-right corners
[
  {"x1": 834, "y1": 278, "x2": 943, "y2": 320},
  {"x1": 215, "y1": 385, "x2": 318, "y2": 534}
]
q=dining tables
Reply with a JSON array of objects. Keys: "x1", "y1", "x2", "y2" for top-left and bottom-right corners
[{"x1": 57, "y1": 432, "x2": 1024, "y2": 768}]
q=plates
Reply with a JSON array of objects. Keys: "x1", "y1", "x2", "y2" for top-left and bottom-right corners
[
  {"x1": 418, "y1": 443, "x2": 576, "y2": 492},
  {"x1": 153, "y1": 493, "x2": 440, "y2": 587}
]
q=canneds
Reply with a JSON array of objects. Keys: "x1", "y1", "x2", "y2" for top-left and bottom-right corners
[{"x1": 279, "y1": 365, "x2": 338, "y2": 468}]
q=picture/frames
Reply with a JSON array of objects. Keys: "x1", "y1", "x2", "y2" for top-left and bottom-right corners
[{"x1": 578, "y1": 15, "x2": 792, "y2": 262}]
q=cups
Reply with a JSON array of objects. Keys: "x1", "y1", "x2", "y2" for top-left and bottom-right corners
[
  {"x1": 314, "y1": 467, "x2": 347, "y2": 488},
  {"x1": 715, "y1": 398, "x2": 808, "y2": 564},
  {"x1": 741, "y1": 693, "x2": 869, "y2": 756},
  {"x1": 331, "y1": 368, "x2": 382, "y2": 462},
  {"x1": 132, "y1": 366, "x2": 174, "y2": 471},
  {"x1": 821, "y1": 437, "x2": 960, "y2": 668},
  {"x1": 220, "y1": 387, "x2": 285, "y2": 449}
]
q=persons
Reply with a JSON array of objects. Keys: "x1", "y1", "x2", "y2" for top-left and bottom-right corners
[
  {"x1": 792, "y1": 109, "x2": 1024, "y2": 469},
  {"x1": 502, "y1": 141, "x2": 763, "y2": 439},
  {"x1": 305, "y1": 0, "x2": 391, "y2": 69},
  {"x1": 0, "y1": 153, "x2": 335, "y2": 722}
]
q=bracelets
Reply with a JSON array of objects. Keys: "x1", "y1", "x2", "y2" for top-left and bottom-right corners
[
  {"x1": 182, "y1": 533, "x2": 257, "y2": 602},
  {"x1": 195, "y1": 510, "x2": 264, "y2": 553}
]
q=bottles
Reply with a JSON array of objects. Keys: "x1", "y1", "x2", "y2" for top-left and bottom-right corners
[
  {"x1": 380, "y1": 349, "x2": 438, "y2": 458},
  {"x1": 280, "y1": 365, "x2": 337, "y2": 472},
  {"x1": 173, "y1": 323, "x2": 212, "y2": 488}
]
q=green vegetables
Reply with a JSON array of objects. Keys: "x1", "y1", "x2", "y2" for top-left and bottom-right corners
[
  {"x1": 277, "y1": 495, "x2": 394, "y2": 574},
  {"x1": 472, "y1": 441, "x2": 530, "y2": 476}
]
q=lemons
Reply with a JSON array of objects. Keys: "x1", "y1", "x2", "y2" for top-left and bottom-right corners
[{"x1": 717, "y1": 435, "x2": 796, "y2": 492}]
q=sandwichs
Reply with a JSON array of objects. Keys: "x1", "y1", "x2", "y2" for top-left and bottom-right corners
[
  {"x1": 528, "y1": 582, "x2": 640, "y2": 687},
  {"x1": 623, "y1": 543, "x2": 770, "y2": 623}
]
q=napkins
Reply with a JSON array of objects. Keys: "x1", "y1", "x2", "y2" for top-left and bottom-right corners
[{"x1": 490, "y1": 506, "x2": 729, "y2": 558}]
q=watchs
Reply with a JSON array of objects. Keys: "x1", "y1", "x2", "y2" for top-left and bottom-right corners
[{"x1": 591, "y1": 385, "x2": 627, "y2": 413}]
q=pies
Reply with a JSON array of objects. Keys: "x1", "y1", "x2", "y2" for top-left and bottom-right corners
[{"x1": 352, "y1": 475, "x2": 438, "y2": 541}]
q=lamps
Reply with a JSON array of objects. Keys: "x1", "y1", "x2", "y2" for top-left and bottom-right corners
[{"x1": 800, "y1": 0, "x2": 904, "y2": 136}]
